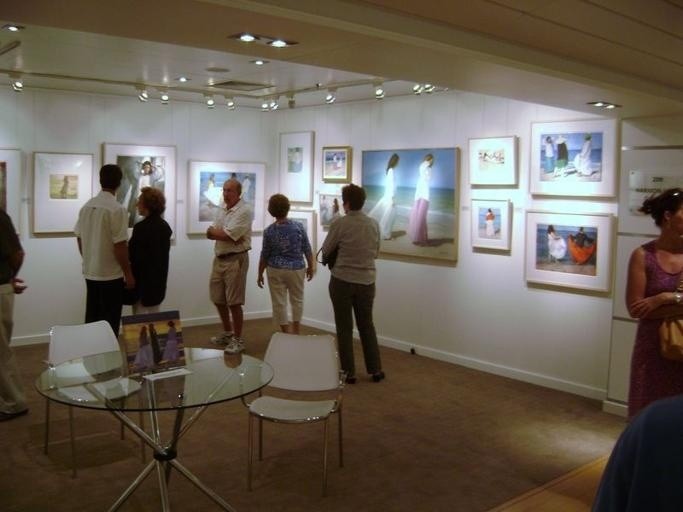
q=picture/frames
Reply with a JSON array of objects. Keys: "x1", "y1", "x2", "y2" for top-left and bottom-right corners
[
  {"x1": 278, "y1": 129, "x2": 316, "y2": 205},
  {"x1": 463, "y1": 116, "x2": 621, "y2": 298},
  {"x1": 187, "y1": 158, "x2": 267, "y2": 239},
  {"x1": 0, "y1": 145, "x2": 22, "y2": 235},
  {"x1": 287, "y1": 209, "x2": 318, "y2": 276},
  {"x1": 31, "y1": 151, "x2": 95, "y2": 235},
  {"x1": 321, "y1": 145, "x2": 353, "y2": 183},
  {"x1": 101, "y1": 143, "x2": 177, "y2": 243}
]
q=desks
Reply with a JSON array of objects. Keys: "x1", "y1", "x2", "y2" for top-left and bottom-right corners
[{"x1": 34, "y1": 346, "x2": 272, "y2": 511}]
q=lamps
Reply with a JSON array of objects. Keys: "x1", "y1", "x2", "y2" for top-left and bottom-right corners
[{"x1": 0, "y1": 69, "x2": 450, "y2": 113}]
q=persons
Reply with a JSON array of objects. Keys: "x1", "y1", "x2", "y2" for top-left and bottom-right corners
[
  {"x1": 547, "y1": 225, "x2": 567, "y2": 263},
  {"x1": 128, "y1": 160, "x2": 163, "y2": 229},
  {"x1": 203, "y1": 172, "x2": 251, "y2": 208},
  {"x1": 590, "y1": 393, "x2": 682, "y2": 512},
  {"x1": 131, "y1": 320, "x2": 179, "y2": 368},
  {"x1": 0, "y1": 208, "x2": 29, "y2": 421},
  {"x1": 408, "y1": 154, "x2": 434, "y2": 247},
  {"x1": 332, "y1": 152, "x2": 342, "y2": 169},
  {"x1": 322, "y1": 181, "x2": 386, "y2": 384},
  {"x1": 366, "y1": 153, "x2": 399, "y2": 240},
  {"x1": 625, "y1": 187, "x2": 683, "y2": 427},
  {"x1": 328, "y1": 198, "x2": 343, "y2": 226},
  {"x1": 126, "y1": 186, "x2": 173, "y2": 315},
  {"x1": 256, "y1": 192, "x2": 315, "y2": 333},
  {"x1": 542, "y1": 134, "x2": 593, "y2": 177},
  {"x1": 568, "y1": 227, "x2": 596, "y2": 264},
  {"x1": 60, "y1": 176, "x2": 69, "y2": 199},
  {"x1": 486, "y1": 209, "x2": 496, "y2": 237},
  {"x1": 206, "y1": 178, "x2": 252, "y2": 354},
  {"x1": 73, "y1": 164, "x2": 136, "y2": 337},
  {"x1": 291, "y1": 147, "x2": 302, "y2": 172}
]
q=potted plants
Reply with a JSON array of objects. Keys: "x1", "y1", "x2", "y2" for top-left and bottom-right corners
[{"x1": 208, "y1": 328, "x2": 247, "y2": 367}]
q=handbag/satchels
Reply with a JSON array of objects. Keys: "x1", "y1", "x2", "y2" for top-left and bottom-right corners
[{"x1": 316, "y1": 244, "x2": 339, "y2": 270}]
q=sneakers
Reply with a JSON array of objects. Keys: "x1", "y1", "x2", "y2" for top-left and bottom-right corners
[
  {"x1": 210, "y1": 329, "x2": 234, "y2": 345},
  {"x1": 222, "y1": 335, "x2": 246, "y2": 355}
]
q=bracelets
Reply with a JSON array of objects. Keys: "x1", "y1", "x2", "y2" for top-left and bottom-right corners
[{"x1": 674, "y1": 291, "x2": 681, "y2": 304}]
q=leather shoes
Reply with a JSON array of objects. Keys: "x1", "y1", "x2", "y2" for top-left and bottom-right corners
[
  {"x1": 0, "y1": 398, "x2": 29, "y2": 421},
  {"x1": 340, "y1": 369, "x2": 359, "y2": 385},
  {"x1": 370, "y1": 371, "x2": 386, "y2": 383}
]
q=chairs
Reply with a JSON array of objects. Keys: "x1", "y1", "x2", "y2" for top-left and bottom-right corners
[
  {"x1": 40, "y1": 319, "x2": 146, "y2": 479},
  {"x1": 238, "y1": 333, "x2": 349, "y2": 497}
]
